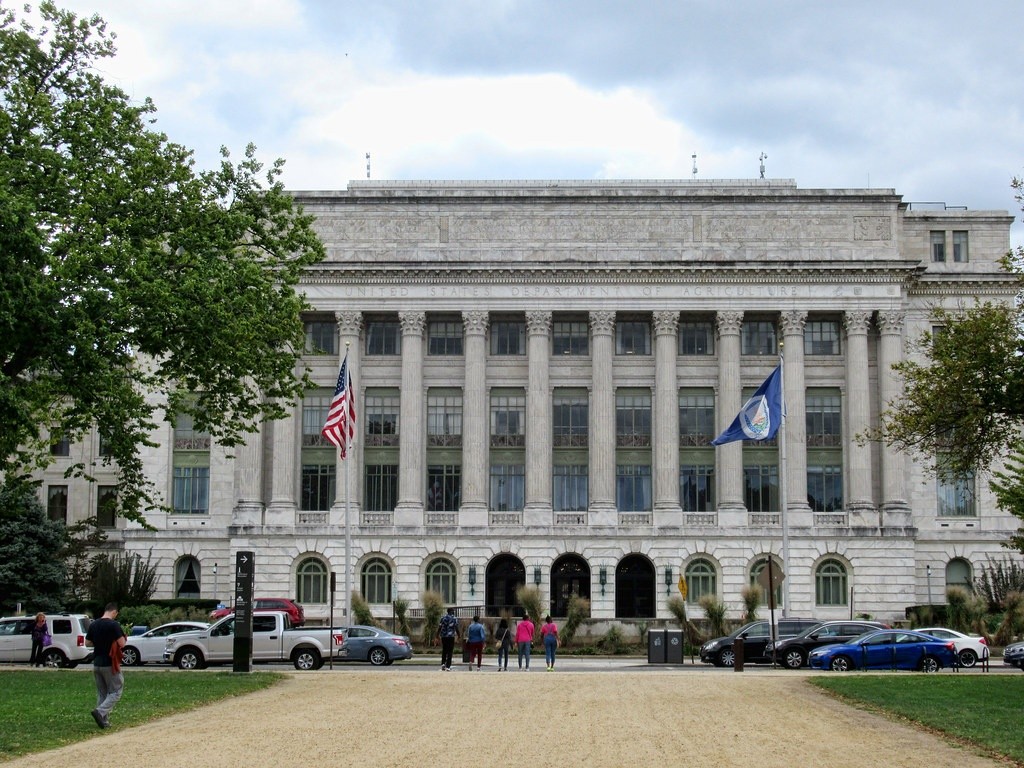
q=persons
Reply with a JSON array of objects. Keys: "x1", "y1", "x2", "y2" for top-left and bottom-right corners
[
  {"x1": 495, "y1": 618, "x2": 513, "y2": 671},
  {"x1": 467, "y1": 616, "x2": 486, "y2": 672},
  {"x1": 29, "y1": 612, "x2": 48, "y2": 667},
  {"x1": 436, "y1": 608, "x2": 461, "y2": 671},
  {"x1": 85, "y1": 603, "x2": 127, "y2": 729},
  {"x1": 515, "y1": 615, "x2": 535, "y2": 671},
  {"x1": 540, "y1": 615, "x2": 561, "y2": 671}
]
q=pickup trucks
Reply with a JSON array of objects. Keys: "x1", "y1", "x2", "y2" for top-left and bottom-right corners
[{"x1": 162, "y1": 611, "x2": 350, "y2": 670}]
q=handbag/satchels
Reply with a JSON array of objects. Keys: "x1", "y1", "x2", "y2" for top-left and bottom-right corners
[
  {"x1": 41, "y1": 627, "x2": 52, "y2": 646},
  {"x1": 496, "y1": 640, "x2": 502, "y2": 649}
]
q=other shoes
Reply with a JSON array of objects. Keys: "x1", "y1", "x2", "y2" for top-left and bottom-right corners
[
  {"x1": 504, "y1": 668, "x2": 508, "y2": 671},
  {"x1": 498, "y1": 668, "x2": 502, "y2": 671},
  {"x1": 525, "y1": 668, "x2": 529, "y2": 672},
  {"x1": 104, "y1": 722, "x2": 110, "y2": 727},
  {"x1": 547, "y1": 667, "x2": 550, "y2": 671},
  {"x1": 446, "y1": 668, "x2": 450, "y2": 671},
  {"x1": 91, "y1": 708, "x2": 104, "y2": 730},
  {"x1": 519, "y1": 669, "x2": 522, "y2": 672},
  {"x1": 469, "y1": 665, "x2": 472, "y2": 670},
  {"x1": 477, "y1": 668, "x2": 480, "y2": 671},
  {"x1": 442, "y1": 666, "x2": 446, "y2": 670},
  {"x1": 551, "y1": 668, "x2": 554, "y2": 671}
]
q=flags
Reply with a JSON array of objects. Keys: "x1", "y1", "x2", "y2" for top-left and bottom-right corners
[
  {"x1": 322, "y1": 353, "x2": 356, "y2": 459},
  {"x1": 710, "y1": 350, "x2": 787, "y2": 447}
]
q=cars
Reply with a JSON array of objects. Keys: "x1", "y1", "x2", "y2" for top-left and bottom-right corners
[
  {"x1": 326, "y1": 625, "x2": 413, "y2": 667},
  {"x1": 210, "y1": 598, "x2": 306, "y2": 628},
  {"x1": 1003, "y1": 641, "x2": 1024, "y2": 672},
  {"x1": 895, "y1": 628, "x2": 990, "y2": 668},
  {"x1": 808, "y1": 629, "x2": 956, "y2": 674},
  {"x1": 764, "y1": 621, "x2": 895, "y2": 669},
  {"x1": 119, "y1": 622, "x2": 212, "y2": 667}
]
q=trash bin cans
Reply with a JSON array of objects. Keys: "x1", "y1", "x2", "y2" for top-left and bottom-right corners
[
  {"x1": 132, "y1": 626, "x2": 148, "y2": 635},
  {"x1": 648, "y1": 629, "x2": 665, "y2": 664},
  {"x1": 665, "y1": 630, "x2": 684, "y2": 664}
]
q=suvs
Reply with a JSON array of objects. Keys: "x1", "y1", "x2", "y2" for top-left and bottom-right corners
[
  {"x1": 0, "y1": 613, "x2": 95, "y2": 669},
  {"x1": 699, "y1": 619, "x2": 837, "y2": 668}
]
